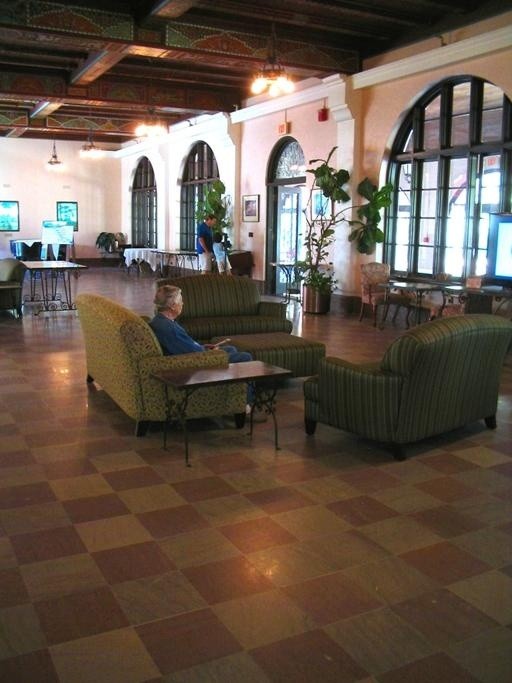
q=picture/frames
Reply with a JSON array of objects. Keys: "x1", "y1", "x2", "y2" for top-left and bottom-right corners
[
  {"x1": 55, "y1": 200, "x2": 79, "y2": 232},
  {"x1": 310, "y1": 189, "x2": 333, "y2": 221},
  {"x1": 0, "y1": 200, "x2": 21, "y2": 233},
  {"x1": 241, "y1": 194, "x2": 259, "y2": 223}
]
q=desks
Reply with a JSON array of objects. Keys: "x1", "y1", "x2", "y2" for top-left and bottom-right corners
[
  {"x1": 20, "y1": 260, "x2": 89, "y2": 317},
  {"x1": 269, "y1": 262, "x2": 304, "y2": 305},
  {"x1": 150, "y1": 251, "x2": 200, "y2": 278},
  {"x1": 378, "y1": 280, "x2": 446, "y2": 331},
  {"x1": 147, "y1": 359, "x2": 293, "y2": 468},
  {"x1": 444, "y1": 283, "x2": 511, "y2": 318},
  {"x1": 123, "y1": 248, "x2": 162, "y2": 278}
]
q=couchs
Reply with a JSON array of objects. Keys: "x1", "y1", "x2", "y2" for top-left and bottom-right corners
[
  {"x1": 154, "y1": 274, "x2": 293, "y2": 340},
  {"x1": 302, "y1": 314, "x2": 510, "y2": 461},
  {"x1": 75, "y1": 292, "x2": 249, "y2": 438},
  {"x1": 0, "y1": 257, "x2": 27, "y2": 325}
]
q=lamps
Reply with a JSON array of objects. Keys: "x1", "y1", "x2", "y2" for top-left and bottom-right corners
[
  {"x1": 250, "y1": 21, "x2": 297, "y2": 98},
  {"x1": 78, "y1": 124, "x2": 105, "y2": 161},
  {"x1": 40, "y1": 139, "x2": 69, "y2": 179}
]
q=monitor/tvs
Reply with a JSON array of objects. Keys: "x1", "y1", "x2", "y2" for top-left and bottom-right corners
[{"x1": 485, "y1": 212, "x2": 512, "y2": 287}]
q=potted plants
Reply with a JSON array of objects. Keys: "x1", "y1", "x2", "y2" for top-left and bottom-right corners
[
  {"x1": 300, "y1": 145, "x2": 394, "y2": 315},
  {"x1": 95, "y1": 230, "x2": 125, "y2": 254}
]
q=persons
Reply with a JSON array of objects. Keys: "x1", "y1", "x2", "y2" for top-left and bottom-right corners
[
  {"x1": 196, "y1": 214, "x2": 216, "y2": 274},
  {"x1": 212, "y1": 231, "x2": 232, "y2": 275},
  {"x1": 149, "y1": 284, "x2": 253, "y2": 415}
]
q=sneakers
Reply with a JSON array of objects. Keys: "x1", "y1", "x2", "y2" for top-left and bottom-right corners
[{"x1": 246, "y1": 404, "x2": 252, "y2": 414}]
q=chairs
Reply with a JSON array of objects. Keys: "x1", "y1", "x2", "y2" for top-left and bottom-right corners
[{"x1": 359, "y1": 262, "x2": 390, "y2": 328}]
